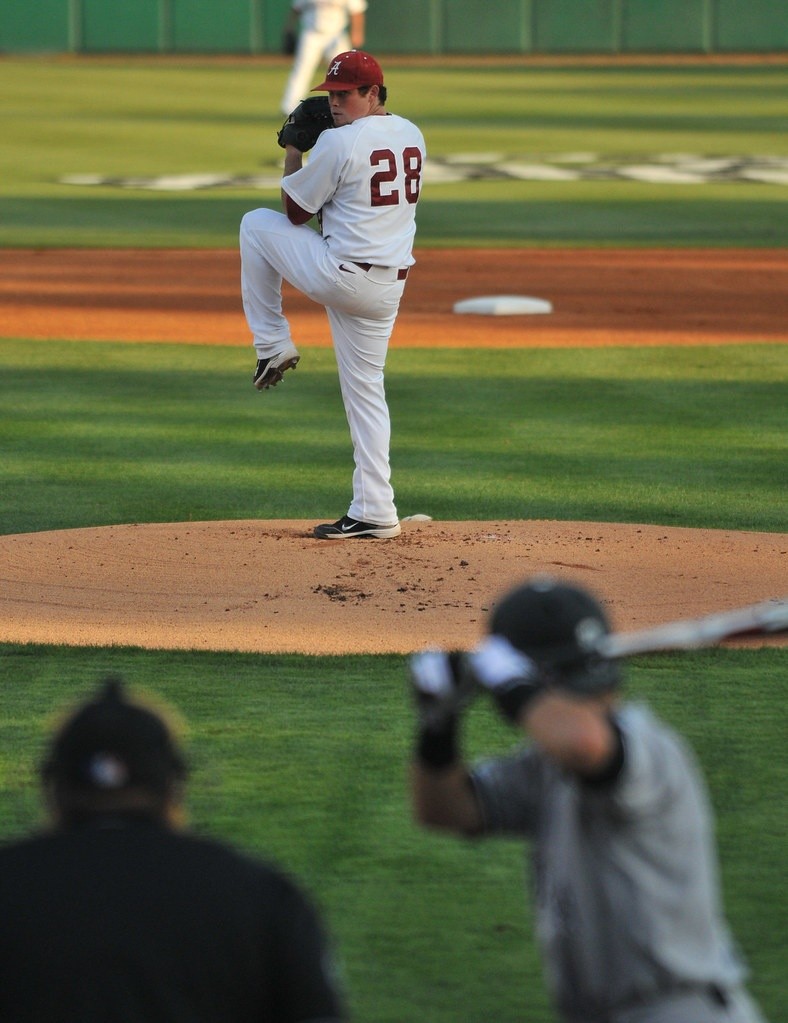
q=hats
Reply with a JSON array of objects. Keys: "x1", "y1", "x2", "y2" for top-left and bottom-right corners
[
  {"x1": 42, "y1": 678, "x2": 186, "y2": 789},
  {"x1": 311, "y1": 50, "x2": 384, "y2": 91},
  {"x1": 491, "y1": 582, "x2": 622, "y2": 692}
]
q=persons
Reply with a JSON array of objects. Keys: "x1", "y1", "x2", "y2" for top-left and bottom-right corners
[
  {"x1": 240, "y1": 50, "x2": 425, "y2": 538},
  {"x1": 283, "y1": 0, "x2": 367, "y2": 116},
  {"x1": 0, "y1": 679, "x2": 351, "y2": 1023},
  {"x1": 408, "y1": 578, "x2": 768, "y2": 1023}
]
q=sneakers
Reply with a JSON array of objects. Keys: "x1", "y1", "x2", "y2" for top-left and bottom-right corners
[
  {"x1": 253, "y1": 346, "x2": 300, "y2": 391},
  {"x1": 314, "y1": 515, "x2": 401, "y2": 539}
]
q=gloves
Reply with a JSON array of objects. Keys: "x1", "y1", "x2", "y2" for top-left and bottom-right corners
[
  {"x1": 409, "y1": 650, "x2": 471, "y2": 734},
  {"x1": 469, "y1": 635, "x2": 544, "y2": 716}
]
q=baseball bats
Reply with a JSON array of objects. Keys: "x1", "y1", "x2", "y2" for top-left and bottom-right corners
[{"x1": 531, "y1": 600, "x2": 788, "y2": 671}]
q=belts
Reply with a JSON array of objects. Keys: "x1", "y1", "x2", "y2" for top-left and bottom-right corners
[{"x1": 353, "y1": 261, "x2": 408, "y2": 280}]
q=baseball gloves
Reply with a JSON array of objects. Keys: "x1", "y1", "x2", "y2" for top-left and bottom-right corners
[{"x1": 277, "y1": 96, "x2": 335, "y2": 152}]
